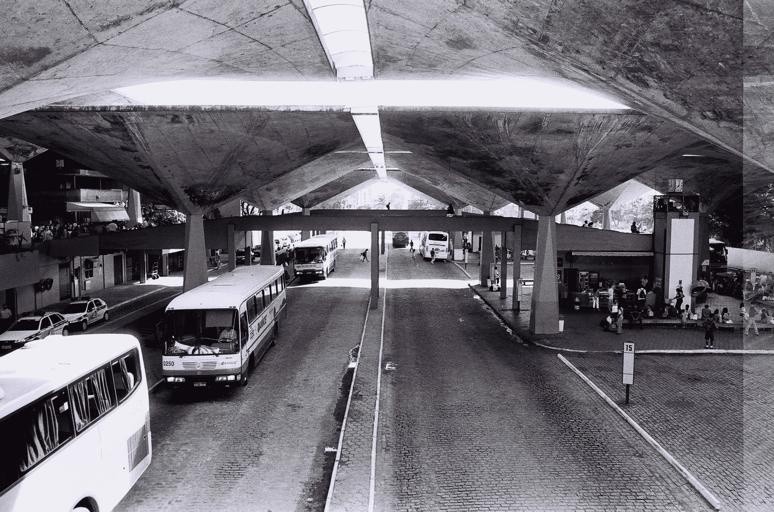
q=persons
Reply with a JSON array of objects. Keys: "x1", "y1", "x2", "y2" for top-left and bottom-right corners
[
  {"x1": 412, "y1": 249, "x2": 415, "y2": 258},
  {"x1": 446, "y1": 203, "x2": 454, "y2": 218},
  {"x1": 340, "y1": 236, "x2": 346, "y2": 249},
  {"x1": 409, "y1": 240, "x2": 414, "y2": 251},
  {"x1": 588, "y1": 222, "x2": 593, "y2": 227},
  {"x1": 359, "y1": 253, "x2": 363, "y2": 261},
  {"x1": 587, "y1": 263, "x2": 773, "y2": 348},
  {"x1": 494, "y1": 267, "x2": 500, "y2": 284},
  {"x1": 218, "y1": 328, "x2": 238, "y2": 346},
  {"x1": 582, "y1": 221, "x2": 588, "y2": 227},
  {"x1": 0, "y1": 303, "x2": 11, "y2": 330},
  {"x1": 29, "y1": 197, "x2": 187, "y2": 243},
  {"x1": 430, "y1": 247, "x2": 435, "y2": 264},
  {"x1": 362, "y1": 248, "x2": 369, "y2": 262},
  {"x1": 631, "y1": 222, "x2": 639, "y2": 234}
]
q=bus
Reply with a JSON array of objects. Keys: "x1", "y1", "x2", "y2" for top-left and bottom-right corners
[
  {"x1": 418, "y1": 230, "x2": 449, "y2": 263},
  {"x1": 0, "y1": 331, "x2": 155, "y2": 511},
  {"x1": 158, "y1": 263, "x2": 287, "y2": 392},
  {"x1": 392, "y1": 232, "x2": 408, "y2": 248},
  {"x1": 291, "y1": 233, "x2": 338, "y2": 282}
]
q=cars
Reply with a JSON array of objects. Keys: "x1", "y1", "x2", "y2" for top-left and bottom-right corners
[
  {"x1": 61, "y1": 296, "x2": 110, "y2": 331},
  {"x1": 0, "y1": 311, "x2": 69, "y2": 354},
  {"x1": 234, "y1": 231, "x2": 300, "y2": 265}
]
